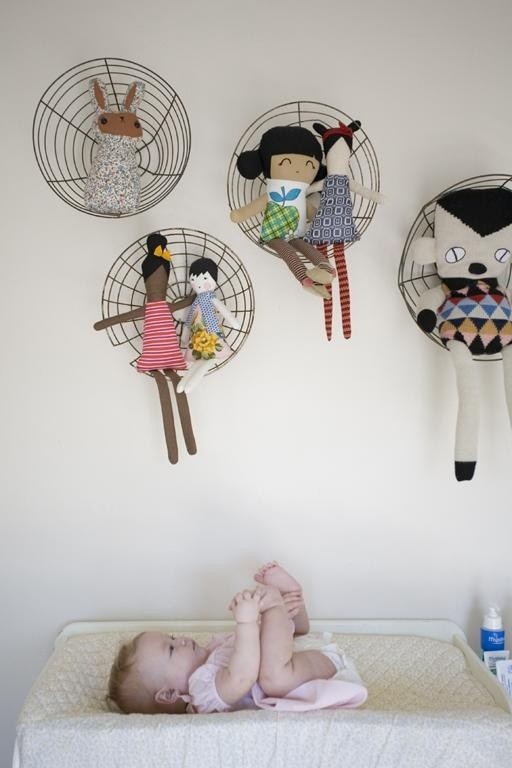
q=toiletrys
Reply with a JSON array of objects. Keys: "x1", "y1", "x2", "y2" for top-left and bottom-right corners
[
  {"x1": 483, "y1": 651, "x2": 509, "y2": 676},
  {"x1": 495, "y1": 660, "x2": 512, "y2": 699}
]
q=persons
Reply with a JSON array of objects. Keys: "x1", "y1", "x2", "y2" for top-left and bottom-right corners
[
  {"x1": 105, "y1": 557, "x2": 367, "y2": 712},
  {"x1": 171, "y1": 256, "x2": 242, "y2": 395},
  {"x1": 229, "y1": 125, "x2": 336, "y2": 301},
  {"x1": 306, "y1": 118, "x2": 386, "y2": 339},
  {"x1": 408, "y1": 184, "x2": 511, "y2": 480},
  {"x1": 90, "y1": 234, "x2": 199, "y2": 464}
]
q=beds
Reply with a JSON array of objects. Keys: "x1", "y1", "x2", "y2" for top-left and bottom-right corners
[{"x1": 11, "y1": 618, "x2": 512, "y2": 768}]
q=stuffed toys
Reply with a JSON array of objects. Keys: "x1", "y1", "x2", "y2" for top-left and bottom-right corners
[{"x1": 87, "y1": 76, "x2": 143, "y2": 215}]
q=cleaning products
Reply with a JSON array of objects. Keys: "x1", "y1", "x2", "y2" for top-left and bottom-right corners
[{"x1": 480, "y1": 603, "x2": 505, "y2": 651}]
